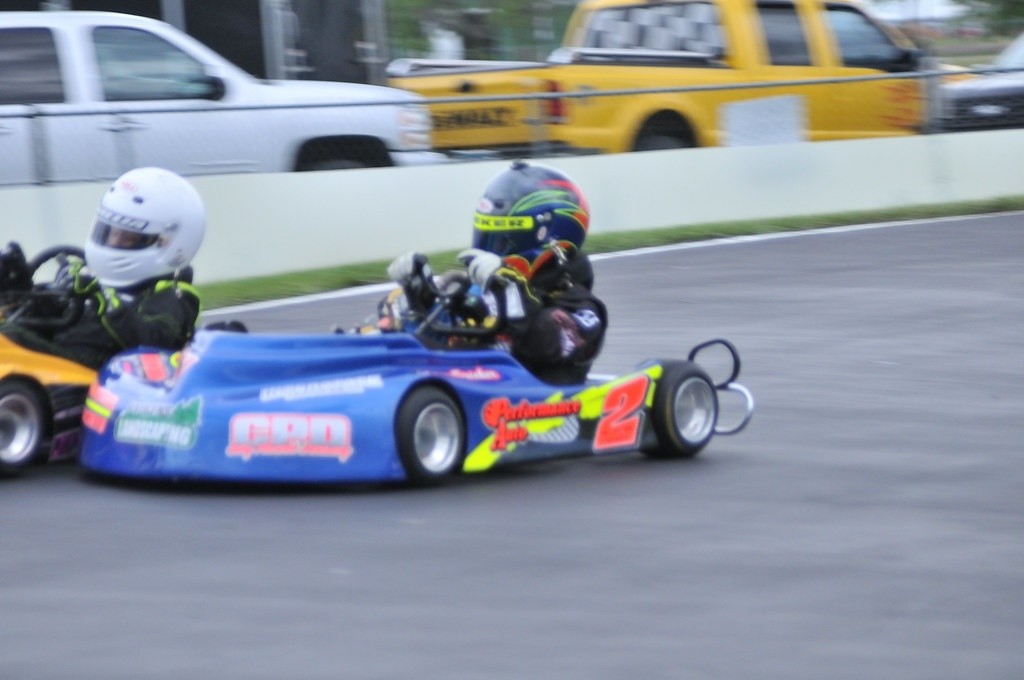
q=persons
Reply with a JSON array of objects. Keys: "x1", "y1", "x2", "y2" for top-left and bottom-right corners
[
  {"x1": 387, "y1": 165, "x2": 607, "y2": 385},
  {"x1": 0, "y1": 167, "x2": 205, "y2": 356}
]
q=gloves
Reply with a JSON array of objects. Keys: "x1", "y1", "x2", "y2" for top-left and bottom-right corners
[
  {"x1": 385, "y1": 251, "x2": 434, "y2": 289},
  {"x1": 457, "y1": 247, "x2": 503, "y2": 295},
  {"x1": 61, "y1": 264, "x2": 102, "y2": 299},
  {"x1": 1, "y1": 240, "x2": 28, "y2": 281}
]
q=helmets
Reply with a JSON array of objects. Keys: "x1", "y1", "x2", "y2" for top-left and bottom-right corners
[
  {"x1": 472, "y1": 163, "x2": 589, "y2": 264},
  {"x1": 84, "y1": 167, "x2": 208, "y2": 289}
]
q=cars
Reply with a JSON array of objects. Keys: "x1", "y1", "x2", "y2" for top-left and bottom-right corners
[{"x1": 0, "y1": 11, "x2": 433, "y2": 185}]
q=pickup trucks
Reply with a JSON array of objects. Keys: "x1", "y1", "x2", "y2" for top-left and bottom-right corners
[{"x1": 386, "y1": 0, "x2": 928, "y2": 154}]
